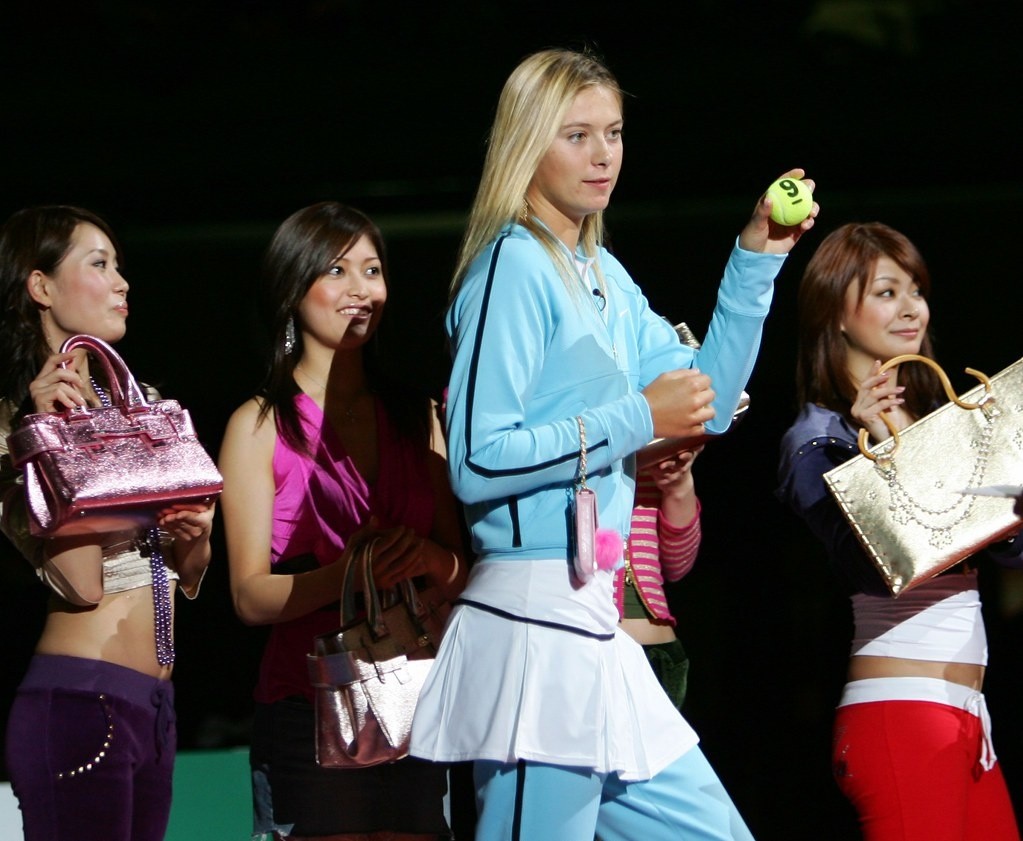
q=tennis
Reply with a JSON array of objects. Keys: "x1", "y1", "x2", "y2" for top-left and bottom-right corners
[{"x1": 764, "y1": 177, "x2": 814, "y2": 227}]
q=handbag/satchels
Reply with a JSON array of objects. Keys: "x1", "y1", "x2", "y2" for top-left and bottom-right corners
[
  {"x1": 822, "y1": 354, "x2": 1023, "y2": 600},
  {"x1": 5, "y1": 334, "x2": 223, "y2": 544},
  {"x1": 307, "y1": 527, "x2": 445, "y2": 769}
]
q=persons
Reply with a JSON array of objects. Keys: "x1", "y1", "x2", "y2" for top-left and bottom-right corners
[
  {"x1": 775, "y1": 224, "x2": 1020, "y2": 841},
  {"x1": 219, "y1": 204, "x2": 476, "y2": 841},
  {"x1": 0, "y1": 208, "x2": 214, "y2": 841},
  {"x1": 407, "y1": 50, "x2": 821, "y2": 841}
]
